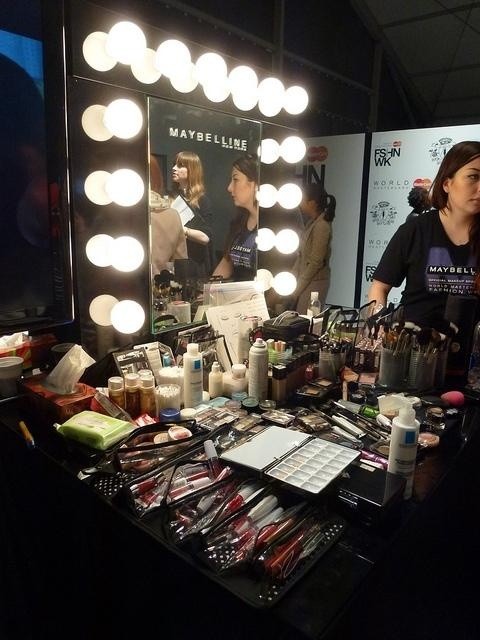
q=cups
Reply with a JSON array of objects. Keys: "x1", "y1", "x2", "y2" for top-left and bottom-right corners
[
  {"x1": 377, "y1": 347, "x2": 449, "y2": 389},
  {"x1": 317, "y1": 349, "x2": 348, "y2": 386},
  {"x1": 39, "y1": 342, "x2": 75, "y2": 376},
  {"x1": 0, "y1": 356, "x2": 24, "y2": 399}
]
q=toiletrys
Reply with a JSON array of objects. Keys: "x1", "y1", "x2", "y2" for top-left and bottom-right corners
[
  {"x1": 306, "y1": 291, "x2": 322, "y2": 317},
  {"x1": 103, "y1": 338, "x2": 288, "y2": 421}
]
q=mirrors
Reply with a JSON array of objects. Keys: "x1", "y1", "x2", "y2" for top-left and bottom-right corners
[{"x1": 145, "y1": 93, "x2": 261, "y2": 336}]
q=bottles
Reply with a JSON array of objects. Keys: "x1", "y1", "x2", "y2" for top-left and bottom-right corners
[
  {"x1": 382, "y1": 399, "x2": 419, "y2": 501},
  {"x1": 106, "y1": 367, "x2": 156, "y2": 420},
  {"x1": 306, "y1": 290, "x2": 322, "y2": 336},
  {"x1": 248, "y1": 339, "x2": 268, "y2": 397},
  {"x1": 208, "y1": 362, "x2": 225, "y2": 399}
]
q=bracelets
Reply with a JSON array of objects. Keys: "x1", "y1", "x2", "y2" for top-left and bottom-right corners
[{"x1": 184, "y1": 227, "x2": 190, "y2": 239}]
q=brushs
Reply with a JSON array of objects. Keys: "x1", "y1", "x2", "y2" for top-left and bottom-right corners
[
  {"x1": 316, "y1": 336, "x2": 346, "y2": 383},
  {"x1": 309, "y1": 399, "x2": 390, "y2": 443},
  {"x1": 376, "y1": 315, "x2": 457, "y2": 386},
  {"x1": 152, "y1": 268, "x2": 184, "y2": 318}
]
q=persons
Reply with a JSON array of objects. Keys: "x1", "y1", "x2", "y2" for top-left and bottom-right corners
[
  {"x1": 365, "y1": 140, "x2": 480, "y2": 372},
  {"x1": 194, "y1": 155, "x2": 259, "y2": 303},
  {"x1": 404, "y1": 185, "x2": 432, "y2": 222},
  {"x1": 285, "y1": 179, "x2": 337, "y2": 315},
  {"x1": 149, "y1": 155, "x2": 189, "y2": 274},
  {"x1": 162, "y1": 150, "x2": 216, "y2": 293}
]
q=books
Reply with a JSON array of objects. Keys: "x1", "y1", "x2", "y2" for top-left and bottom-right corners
[{"x1": 169, "y1": 194, "x2": 196, "y2": 227}]
